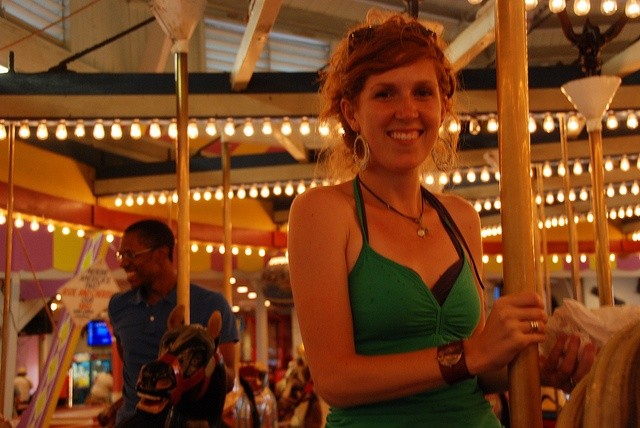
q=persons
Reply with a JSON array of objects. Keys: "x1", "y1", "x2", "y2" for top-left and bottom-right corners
[
  {"x1": 12, "y1": 367, "x2": 33, "y2": 417},
  {"x1": 85, "y1": 369, "x2": 113, "y2": 406},
  {"x1": 286, "y1": 6, "x2": 597, "y2": 428},
  {"x1": 108, "y1": 219, "x2": 240, "y2": 428}
]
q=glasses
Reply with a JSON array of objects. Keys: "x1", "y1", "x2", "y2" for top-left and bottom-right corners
[
  {"x1": 347, "y1": 23, "x2": 437, "y2": 56},
  {"x1": 119, "y1": 249, "x2": 152, "y2": 261}
]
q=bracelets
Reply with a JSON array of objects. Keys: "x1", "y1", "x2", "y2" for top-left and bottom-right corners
[{"x1": 437, "y1": 339, "x2": 475, "y2": 387}]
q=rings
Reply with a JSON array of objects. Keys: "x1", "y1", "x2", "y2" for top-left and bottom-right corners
[{"x1": 530, "y1": 320, "x2": 538, "y2": 333}]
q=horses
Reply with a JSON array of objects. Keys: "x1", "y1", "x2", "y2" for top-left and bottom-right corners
[{"x1": 135, "y1": 304, "x2": 236, "y2": 428}]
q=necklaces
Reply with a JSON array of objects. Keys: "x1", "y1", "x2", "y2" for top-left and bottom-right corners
[{"x1": 358, "y1": 172, "x2": 426, "y2": 238}]
